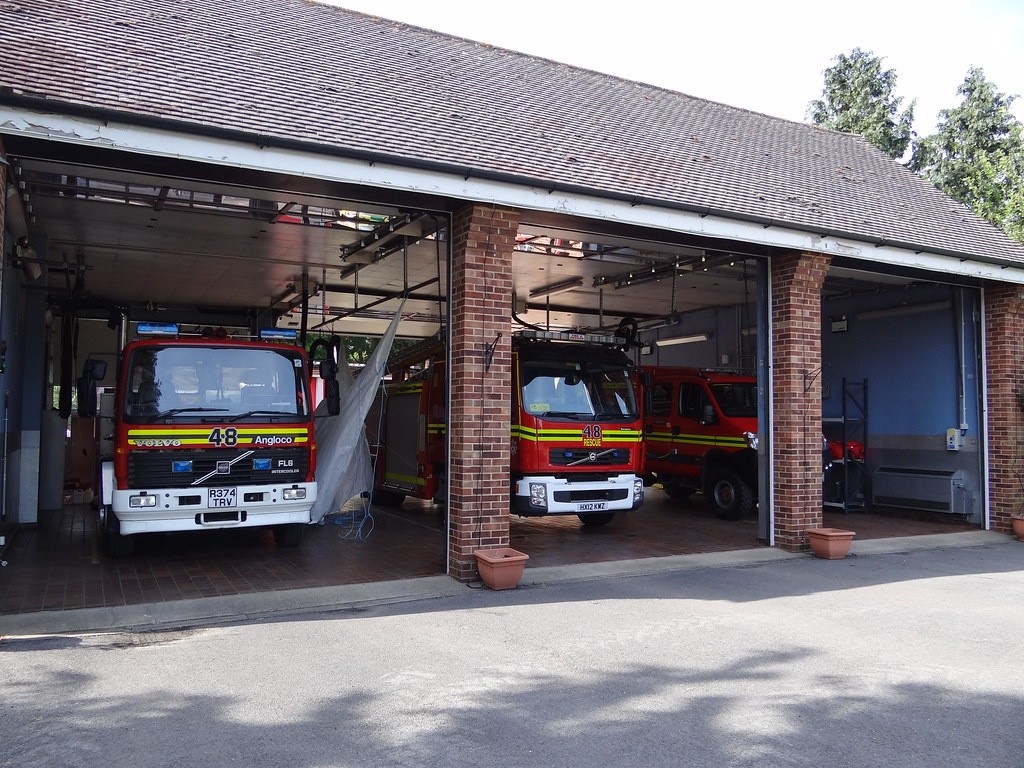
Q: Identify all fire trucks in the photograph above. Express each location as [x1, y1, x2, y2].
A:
[77, 323, 341, 559]
[352, 316, 643, 527]
[600, 364, 832, 521]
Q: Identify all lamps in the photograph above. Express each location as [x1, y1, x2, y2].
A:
[30, 216, 35, 224]
[529, 281, 582, 298]
[601, 255, 735, 285]
[15, 166, 21, 176]
[12, 234, 29, 268]
[19, 180, 27, 191]
[26, 204, 33, 213]
[344, 214, 412, 254]
[24, 193, 31, 203]
[341, 263, 368, 282]
[826, 313, 848, 334]
[655, 333, 709, 346]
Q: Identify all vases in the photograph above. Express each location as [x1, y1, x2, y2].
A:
[806, 528, 855, 558]
[473, 546, 529, 591]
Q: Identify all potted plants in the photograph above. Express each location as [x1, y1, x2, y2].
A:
[1011, 389, 1024, 542]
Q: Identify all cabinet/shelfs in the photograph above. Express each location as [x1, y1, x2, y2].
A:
[822, 379, 868, 512]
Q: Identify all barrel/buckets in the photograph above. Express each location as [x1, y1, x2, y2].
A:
[63, 488, 94, 505]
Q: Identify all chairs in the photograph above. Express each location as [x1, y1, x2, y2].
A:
[239, 368, 278, 403]
[139, 367, 181, 408]
[522, 364, 542, 406]
